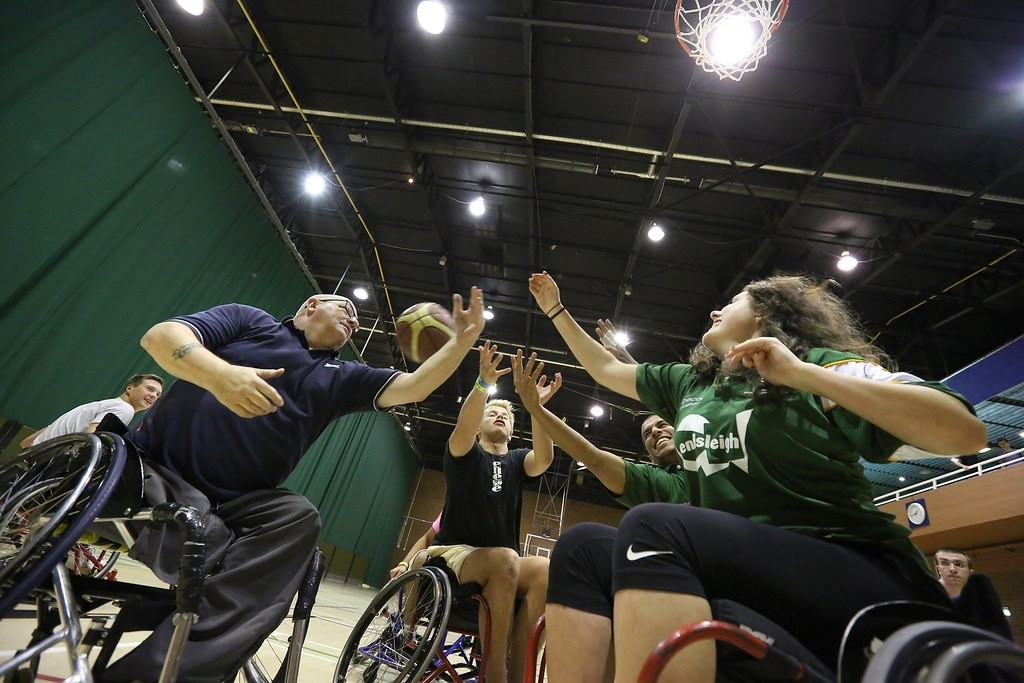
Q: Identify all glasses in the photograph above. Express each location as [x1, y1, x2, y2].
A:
[305, 298, 360, 333]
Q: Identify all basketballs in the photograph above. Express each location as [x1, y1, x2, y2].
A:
[395, 301, 457, 365]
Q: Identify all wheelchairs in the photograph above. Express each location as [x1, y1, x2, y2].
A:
[523, 593, 1024, 683]
[332, 555, 512, 683]
[0, 412, 325, 683]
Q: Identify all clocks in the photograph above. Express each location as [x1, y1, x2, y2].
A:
[907, 503, 926, 525]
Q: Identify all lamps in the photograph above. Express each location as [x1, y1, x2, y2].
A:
[469, 192, 485, 216]
[837, 248, 858, 271]
[647, 222, 665, 241]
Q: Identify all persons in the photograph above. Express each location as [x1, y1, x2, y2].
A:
[998, 438, 1024, 468]
[596, 317, 638, 365]
[93, 287, 484, 683]
[390, 511, 443, 642]
[951, 455, 979, 479]
[20, 374, 164, 449]
[528, 272, 988, 683]
[509, 348, 690, 510]
[427, 340, 563, 683]
[934, 548, 1014, 682]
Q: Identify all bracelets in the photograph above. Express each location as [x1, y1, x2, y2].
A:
[474, 377, 494, 393]
[543, 302, 564, 319]
[398, 562, 408, 568]
